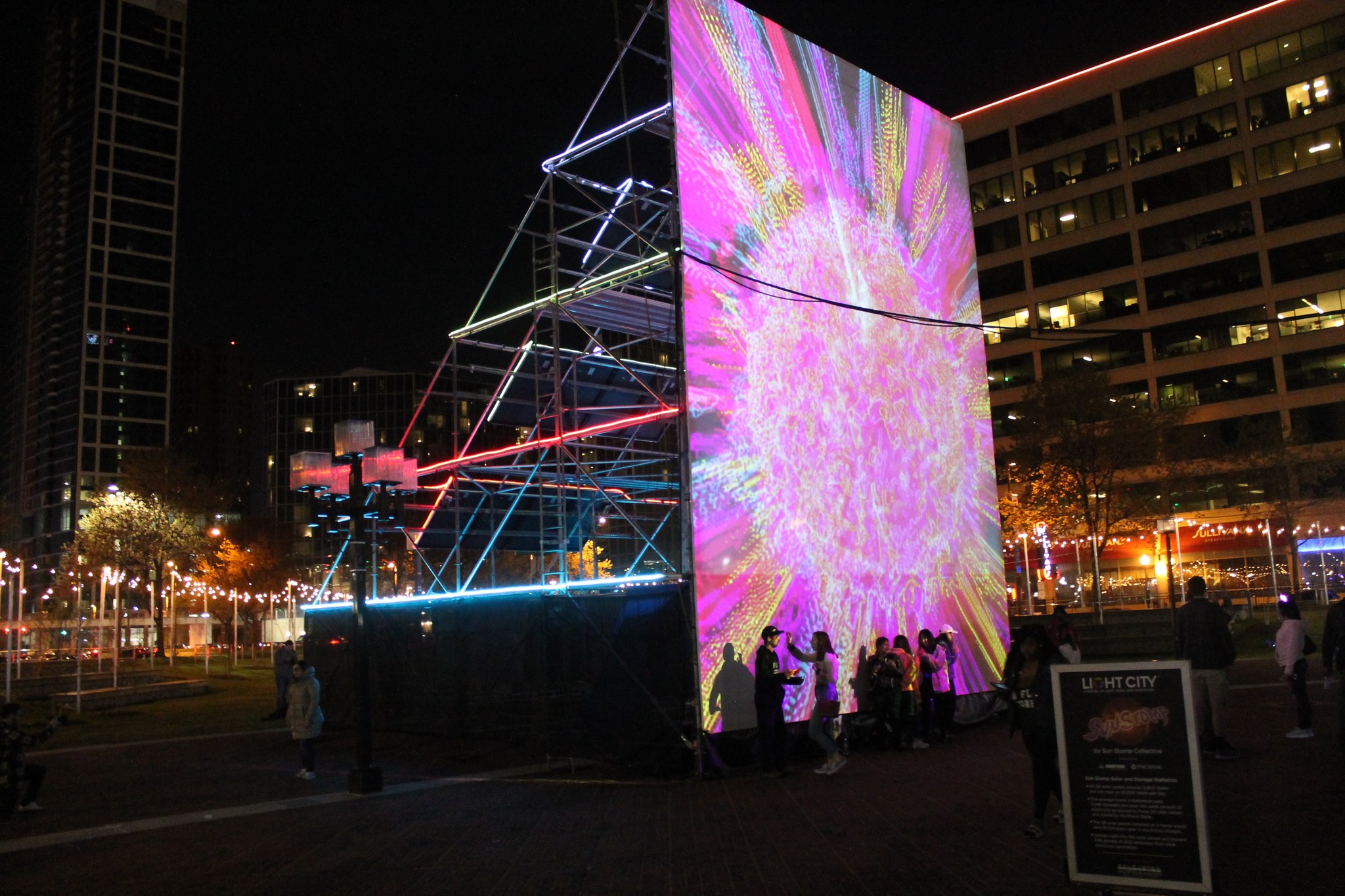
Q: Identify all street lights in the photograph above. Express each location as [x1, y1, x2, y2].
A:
[290, 420, 419, 794]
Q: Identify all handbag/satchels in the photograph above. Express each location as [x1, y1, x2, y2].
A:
[1301, 635, 1317, 655]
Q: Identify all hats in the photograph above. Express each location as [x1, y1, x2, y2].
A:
[939, 624, 958, 634]
[762, 626, 783, 639]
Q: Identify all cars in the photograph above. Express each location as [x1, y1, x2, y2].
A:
[1279, 590, 1338, 602]
[119, 649, 151, 659]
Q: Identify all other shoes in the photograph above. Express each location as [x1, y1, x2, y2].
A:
[815, 764, 827, 775]
[826, 754, 847, 775]
[1198, 740, 1212, 754]
[1053, 808, 1064, 825]
[912, 739, 929, 749]
[297, 769, 317, 781]
[1027, 823, 1044, 838]
[1212, 746, 1238, 758]
[1284, 726, 1314, 738]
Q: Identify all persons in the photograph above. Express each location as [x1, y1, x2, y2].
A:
[1273, 599, 1316, 738]
[284, 660, 323, 780]
[1322, 594, 1345, 696]
[1173, 576, 1242, 759]
[753, 605, 1082, 836]
[272, 640, 298, 723]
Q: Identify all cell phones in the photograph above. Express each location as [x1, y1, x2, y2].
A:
[990, 681, 1009, 689]
